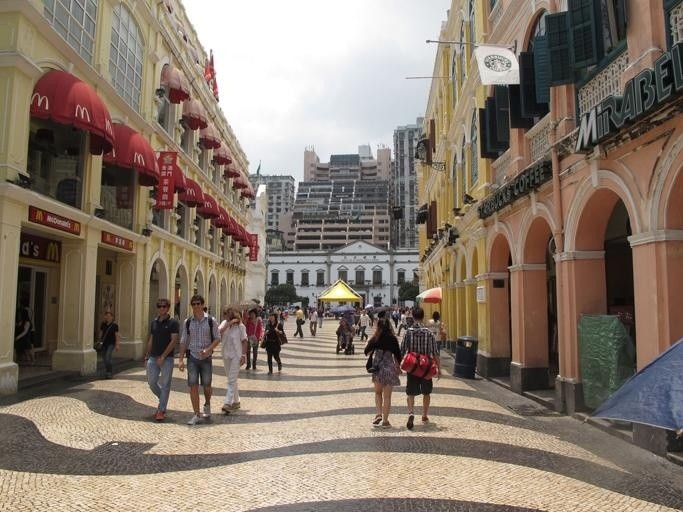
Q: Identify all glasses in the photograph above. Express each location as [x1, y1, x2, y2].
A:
[157, 305, 167, 308]
[191, 303, 200, 306]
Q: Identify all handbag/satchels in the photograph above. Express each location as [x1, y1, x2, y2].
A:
[400, 351, 437, 381]
[301, 321, 305, 325]
[365, 356, 380, 372]
[260, 334, 268, 349]
[440, 322, 448, 339]
[94, 338, 103, 352]
[248, 335, 258, 348]
[278, 331, 287, 345]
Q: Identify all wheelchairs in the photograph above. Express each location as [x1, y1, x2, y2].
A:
[335, 330, 355, 356]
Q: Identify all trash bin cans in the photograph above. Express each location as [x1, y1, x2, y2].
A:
[453, 336, 478, 379]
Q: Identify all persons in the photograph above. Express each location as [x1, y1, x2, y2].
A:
[396, 306, 413, 336]
[336, 306, 373, 355]
[425, 312, 447, 360]
[242, 303, 288, 376]
[98, 312, 120, 379]
[394, 307, 441, 429]
[145, 294, 249, 425]
[14, 303, 36, 367]
[363, 311, 401, 428]
[292, 306, 324, 338]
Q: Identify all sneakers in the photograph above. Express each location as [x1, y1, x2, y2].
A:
[106, 376, 112, 379]
[407, 412, 414, 429]
[233, 402, 241, 409]
[246, 364, 282, 376]
[382, 421, 391, 428]
[155, 410, 164, 420]
[422, 416, 429, 423]
[222, 404, 234, 412]
[187, 415, 202, 425]
[203, 403, 212, 418]
[373, 414, 382, 424]
